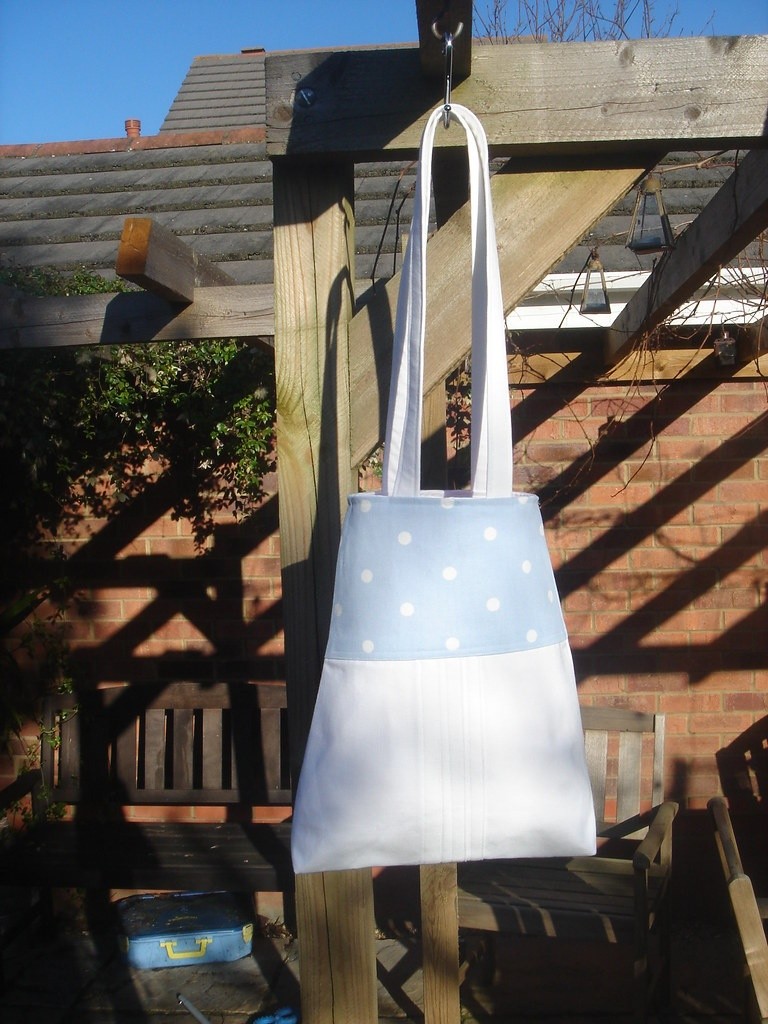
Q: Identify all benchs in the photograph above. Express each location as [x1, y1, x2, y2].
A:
[0, 665, 294, 953]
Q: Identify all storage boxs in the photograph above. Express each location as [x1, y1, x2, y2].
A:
[115, 890, 257, 969]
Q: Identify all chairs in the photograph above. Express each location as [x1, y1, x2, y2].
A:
[457, 704, 679, 1024]
[707, 795, 767, 1024]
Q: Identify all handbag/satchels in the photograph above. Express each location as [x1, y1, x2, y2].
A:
[290, 104, 598, 874]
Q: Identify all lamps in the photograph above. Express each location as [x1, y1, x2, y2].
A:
[578, 249, 611, 314]
[625, 167, 676, 255]
